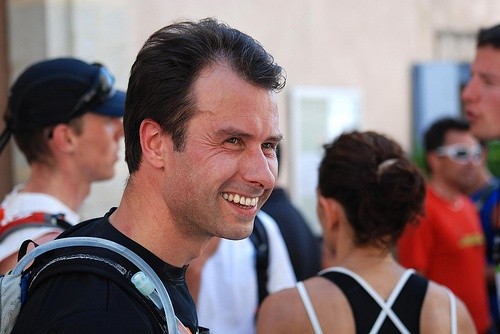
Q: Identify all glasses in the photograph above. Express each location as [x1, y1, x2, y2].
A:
[436, 142, 484, 167]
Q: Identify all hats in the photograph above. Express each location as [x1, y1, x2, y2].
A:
[4, 57, 126, 128]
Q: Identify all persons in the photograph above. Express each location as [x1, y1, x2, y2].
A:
[0, 17, 286, 334]
[0, 57, 126, 275]
[194, 26, 500, 334]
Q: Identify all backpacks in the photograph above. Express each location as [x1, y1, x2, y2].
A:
[0, 239, 190, 334]
